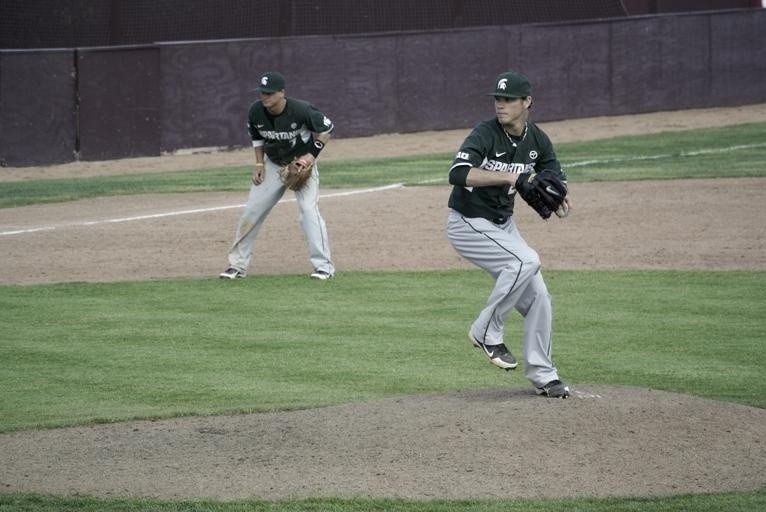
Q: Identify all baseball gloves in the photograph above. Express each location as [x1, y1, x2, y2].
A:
[278, 156, 314, 192]
[515, 169, 567, 219]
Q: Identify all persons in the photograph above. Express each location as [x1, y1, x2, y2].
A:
[218, 70, 335, 280]
[446, 70, 572, 399]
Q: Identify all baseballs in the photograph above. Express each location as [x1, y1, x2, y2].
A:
[555, 202, 569, 217]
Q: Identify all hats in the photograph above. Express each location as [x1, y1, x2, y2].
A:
[484, 70, 535, 100]
[251, 71, 287, 96]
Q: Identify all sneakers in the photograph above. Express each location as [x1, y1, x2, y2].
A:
[533, 376, 571, 400]
[219, 267, 248, 279]
[310, 267, 335, 280]
[467, 324, 519, 371]
